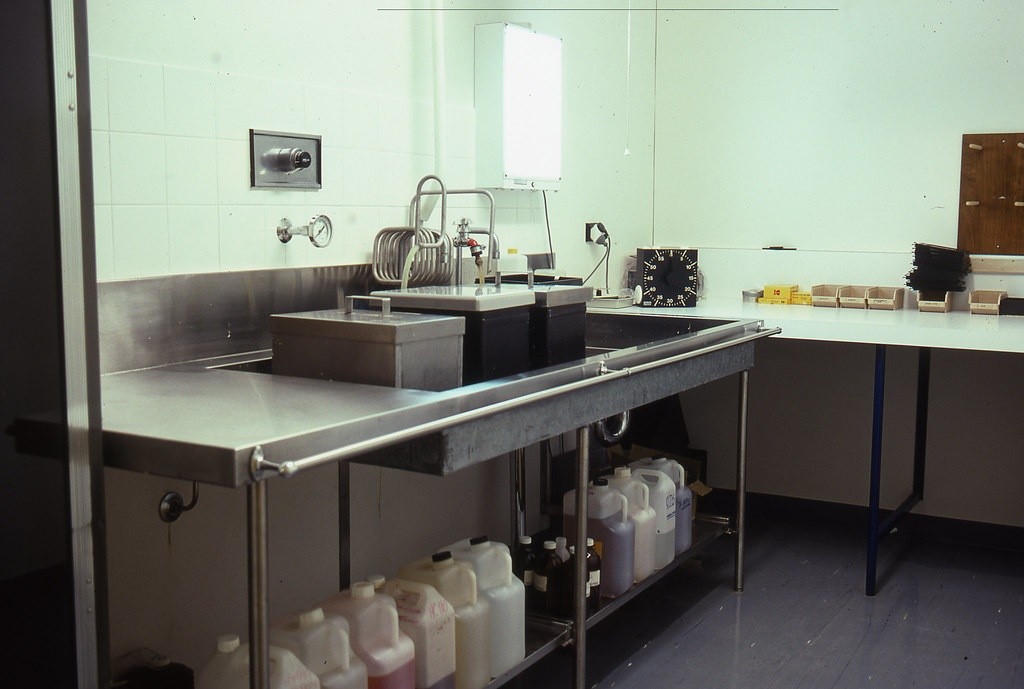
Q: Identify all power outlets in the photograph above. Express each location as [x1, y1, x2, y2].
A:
[586, 222, 600, 242]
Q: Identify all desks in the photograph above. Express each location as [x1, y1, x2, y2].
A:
[584, 297, 1023, 598]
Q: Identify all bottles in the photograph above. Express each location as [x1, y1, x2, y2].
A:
[556, 537, 570, 561]
[645, 458, 692, 556]
[512, 536, 535, 586]
[270, 608, 368, 689]
[533, 541, 562, 602]
[198, 634, 320, 689]
[632, 469, 676, 570]
[591, 467, 656, 582]
[437, 535, 525, 677]
[587, 538, 601, 609]
[398, 551, 491, 689]
[555, 545, 590, 621]
[313, 581, 416, 689]
[564, 478, 635, 599]
[367, 575, 456, 689]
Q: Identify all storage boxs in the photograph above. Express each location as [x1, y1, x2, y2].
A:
[368, 286, 536, 387]
[480, 283, 594, 368]
